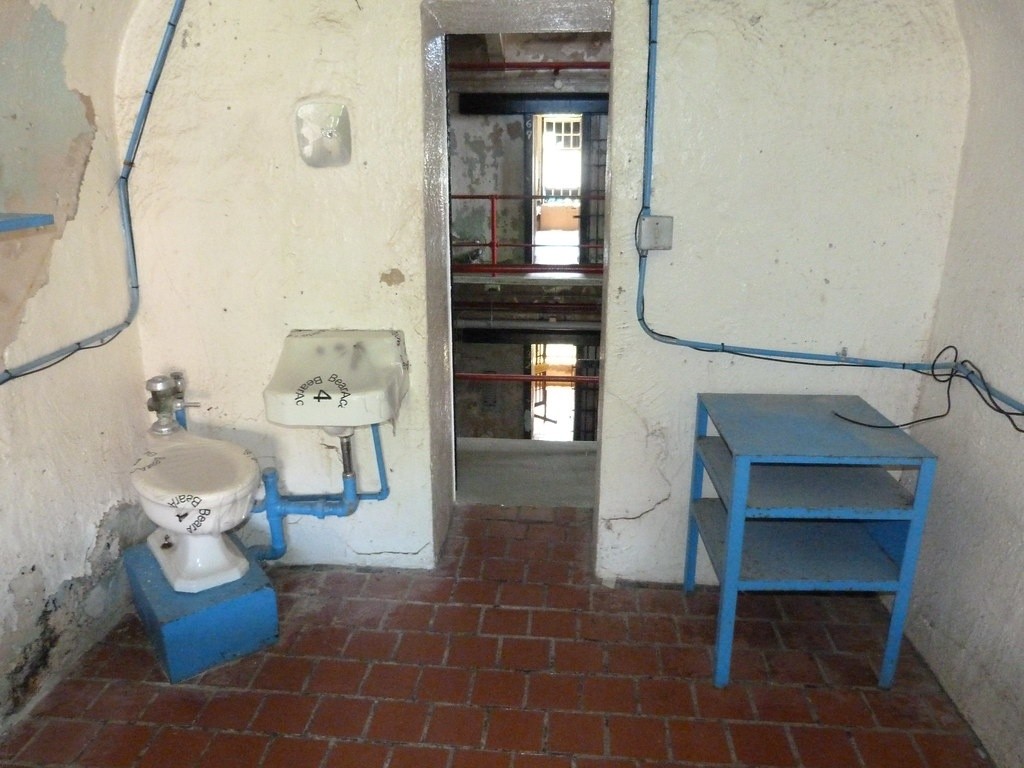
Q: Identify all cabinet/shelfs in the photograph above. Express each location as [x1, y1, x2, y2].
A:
[681, 393, 938, 688]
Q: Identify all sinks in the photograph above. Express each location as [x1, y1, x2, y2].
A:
[129, 424, 266, 593]
[263, 329, 410, 428]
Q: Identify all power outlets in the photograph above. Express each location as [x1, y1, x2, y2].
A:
[639, 214, 674, 252]
